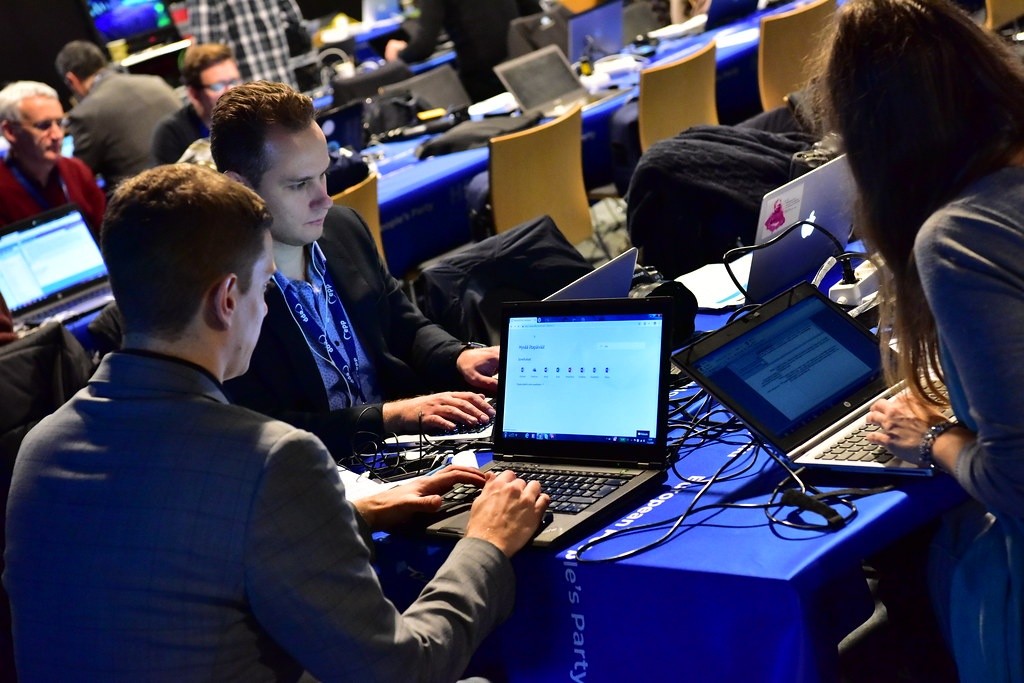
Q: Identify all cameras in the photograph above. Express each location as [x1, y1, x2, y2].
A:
[627, 265, 700, 350]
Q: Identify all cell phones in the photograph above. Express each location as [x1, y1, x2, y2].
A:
[369, 456, 444, 484]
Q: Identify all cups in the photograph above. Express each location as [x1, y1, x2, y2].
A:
[106, 39, 128, 62]
[335, 60, 355, 80]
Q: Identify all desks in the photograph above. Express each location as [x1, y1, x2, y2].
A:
[0, 0, 968, 683]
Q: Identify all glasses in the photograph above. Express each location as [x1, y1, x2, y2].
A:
[190, 77, 245, 95]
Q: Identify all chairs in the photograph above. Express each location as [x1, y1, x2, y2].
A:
[0, 0, 1023, 510]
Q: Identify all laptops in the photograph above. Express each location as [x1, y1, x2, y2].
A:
[314, 0, 760, 153]
[385, 153, 962, 556]
[60, 133, 109, 187]
[0, 205, 119, 326]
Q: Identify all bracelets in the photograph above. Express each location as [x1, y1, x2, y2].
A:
[918, 420, 959, 470]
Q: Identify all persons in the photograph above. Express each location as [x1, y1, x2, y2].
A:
[386, 0, 544, 113]
[152, 44, 244, 168]
[188, 0, 308, 99]
[196, 81, 505, 452]
[825, 0, 1024, 683]
[0, 80, 107, 338]
[0, 165, 552, 683]
[59, 41, 186, 196]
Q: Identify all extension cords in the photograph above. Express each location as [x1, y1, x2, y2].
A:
[829, 251, 890, 305]
